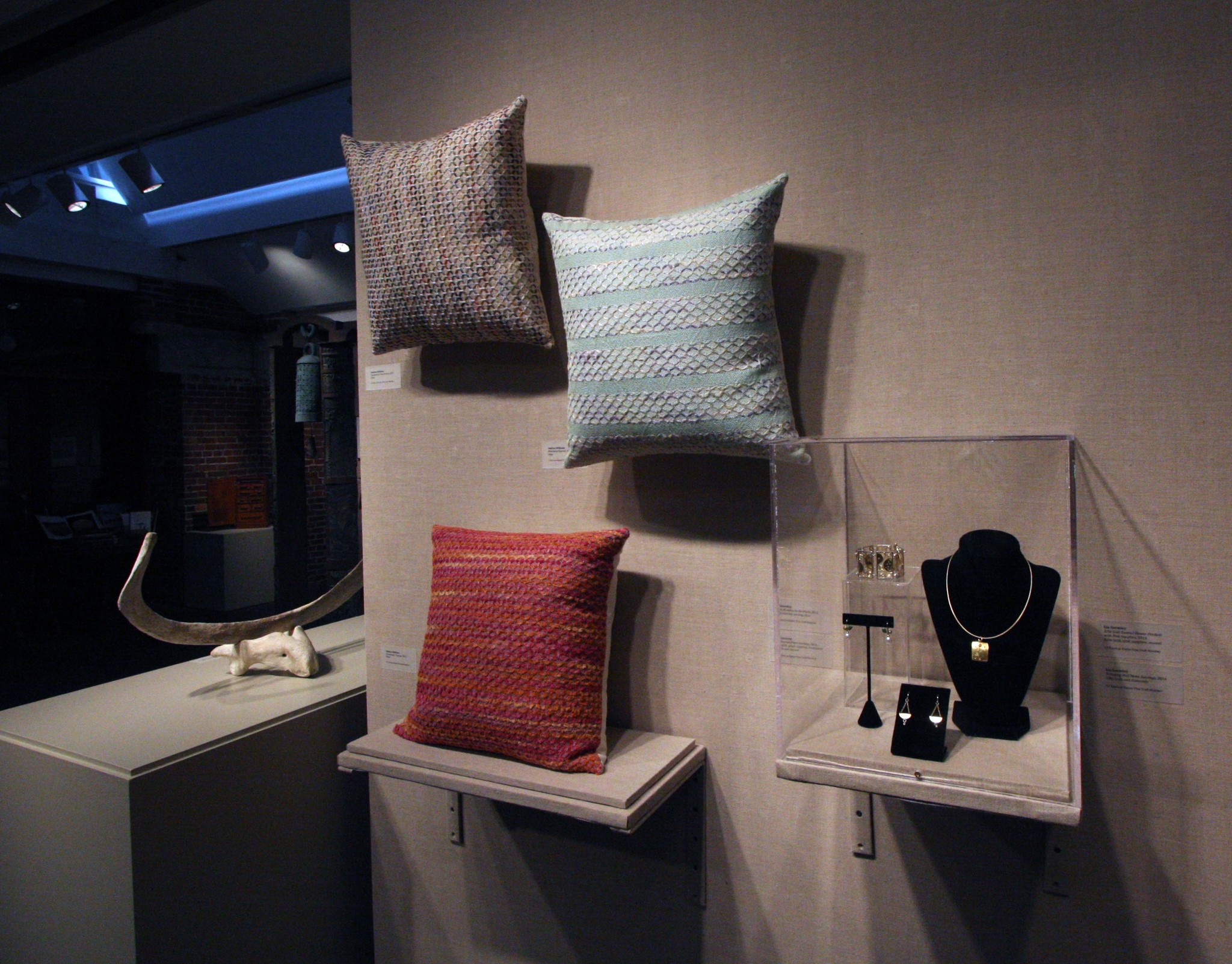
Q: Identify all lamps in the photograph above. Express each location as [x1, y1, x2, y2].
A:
[292, 221, 313, 259]
[118, 148, 164, 194]
[243, 234, 269, 274]
[0, 184, 25, 227]
[333, 216, 351, 254]
[45, 168, 90, 213]
[5, 177, 44, 219]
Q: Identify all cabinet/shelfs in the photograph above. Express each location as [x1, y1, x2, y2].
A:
[0, 609, 371, 964]
[760, 432, 1084, 830]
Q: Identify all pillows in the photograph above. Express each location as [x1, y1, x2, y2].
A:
[339, 99, 556, 352]
[392, 526, 633, 773]
[541, 171, 810, 472]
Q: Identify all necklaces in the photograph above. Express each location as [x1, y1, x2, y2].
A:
[946, 552, 1034, 662]
